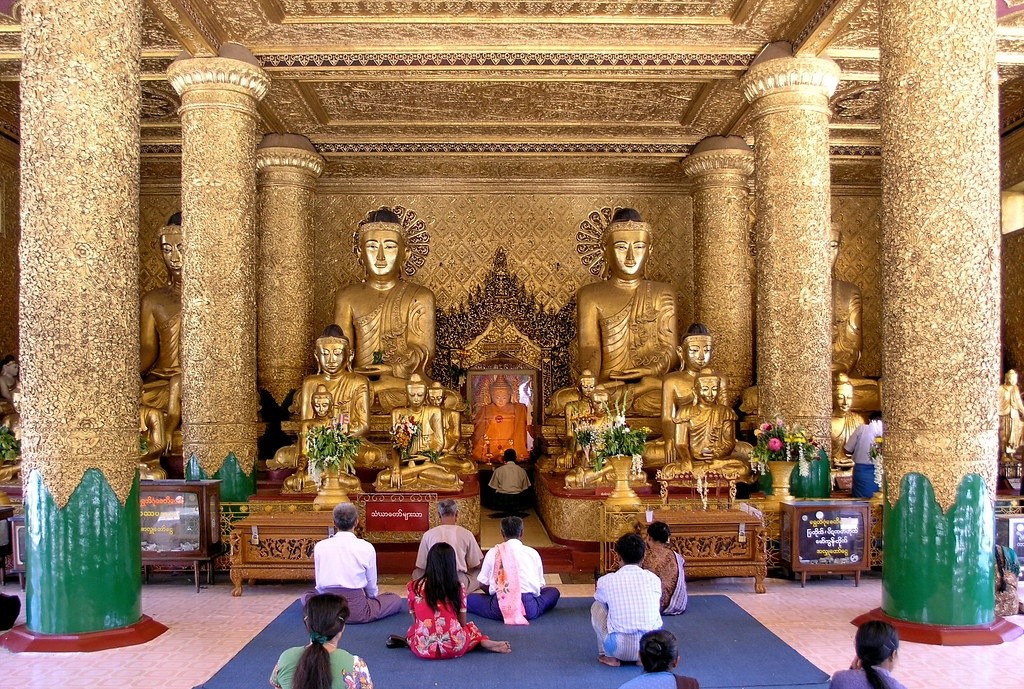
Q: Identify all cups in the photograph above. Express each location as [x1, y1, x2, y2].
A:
[645, 511, 653, 523]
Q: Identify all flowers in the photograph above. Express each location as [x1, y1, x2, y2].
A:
[306, 413, 366, 490]
[867, 435, 884, 490]
[570, 391, 653, 479]
[388, 413, 422, 460]
[746, 417, 822, 478]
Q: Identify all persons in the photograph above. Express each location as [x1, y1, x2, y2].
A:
[473, 373, 529, 462]
[829, 372, 865, 490]
[740, 219, 879, 414]
[0, 353, 19, 423]
[466, 516, 559, 626]
[266, 205, 474, 496]
[0, 385, 22, 485]
[393, 542, 512, 658]
[545, 207, 756, 489]
[412, 498, 483, 595]
[589, 533, 663, 668]
[998, 369, 1024, 455]
[829, 619, 906, 689]
[844, 409, 883, 498]
[617, 629, 701, 689]
[138, 208, 182, 453]
[138, 373, 168, 481]
[299, 502, 403, 623]
[268, 593, 375, 689]
[641, 521, 688, 615]
[486, 448, 533, 512]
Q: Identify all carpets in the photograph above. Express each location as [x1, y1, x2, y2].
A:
[193, 594, 836, 689]
